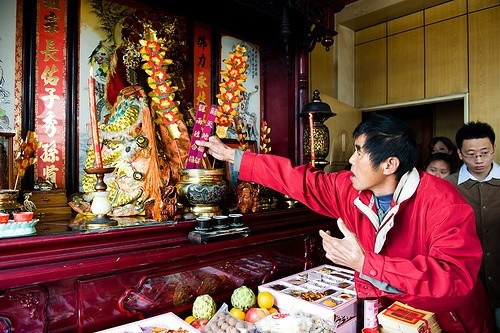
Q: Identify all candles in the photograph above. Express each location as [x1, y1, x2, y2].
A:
[308, 113, 315, 168]
[88, 57, 104, 168]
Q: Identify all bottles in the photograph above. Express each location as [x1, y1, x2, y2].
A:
[357, 298, 378, 333]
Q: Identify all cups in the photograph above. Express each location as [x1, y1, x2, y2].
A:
[195, 214, 244, 231]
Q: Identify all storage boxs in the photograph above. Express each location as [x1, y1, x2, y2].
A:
[258, 264, 357, 328]
[332, 317, 357, 333]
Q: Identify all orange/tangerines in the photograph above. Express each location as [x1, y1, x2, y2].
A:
[230, 307, 246, 321]
[257, 292, 278, 314]
[185, 316, 196, 325]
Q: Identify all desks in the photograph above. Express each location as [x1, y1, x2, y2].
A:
[0, 204, 353, 333]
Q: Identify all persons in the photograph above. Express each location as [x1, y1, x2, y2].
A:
[425, 120, 500, 333]
[195, 114, 494, 333]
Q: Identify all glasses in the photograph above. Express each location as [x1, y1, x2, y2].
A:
[460, 146, 495, 159]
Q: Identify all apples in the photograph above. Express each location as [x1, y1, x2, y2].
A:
[244, 307, 270, 323]
